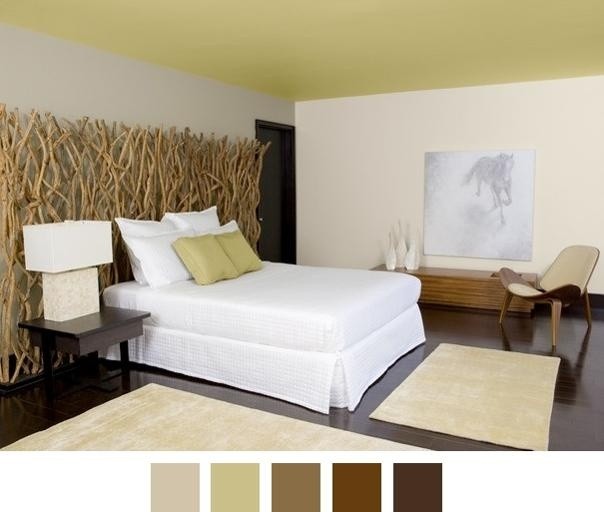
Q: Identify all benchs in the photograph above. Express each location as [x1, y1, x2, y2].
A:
[369, 263, 537, 314]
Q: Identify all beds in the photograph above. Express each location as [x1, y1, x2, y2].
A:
[105, 260, 426, 415]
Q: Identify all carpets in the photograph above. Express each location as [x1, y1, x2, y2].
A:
[0, 382, 437, 451]
[367, 344, 562, 450]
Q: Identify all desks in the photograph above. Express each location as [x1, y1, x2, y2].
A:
[18, 304, 150, 411]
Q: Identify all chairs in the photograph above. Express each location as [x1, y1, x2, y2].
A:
[495, 243, 600, 346]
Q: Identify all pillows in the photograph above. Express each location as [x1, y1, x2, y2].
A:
[112, 205, 264, 288]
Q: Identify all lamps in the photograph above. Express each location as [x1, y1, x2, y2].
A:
[22, 220, 114, 324]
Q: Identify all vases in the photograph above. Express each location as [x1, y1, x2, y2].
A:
[385, 218, 422, 270]
